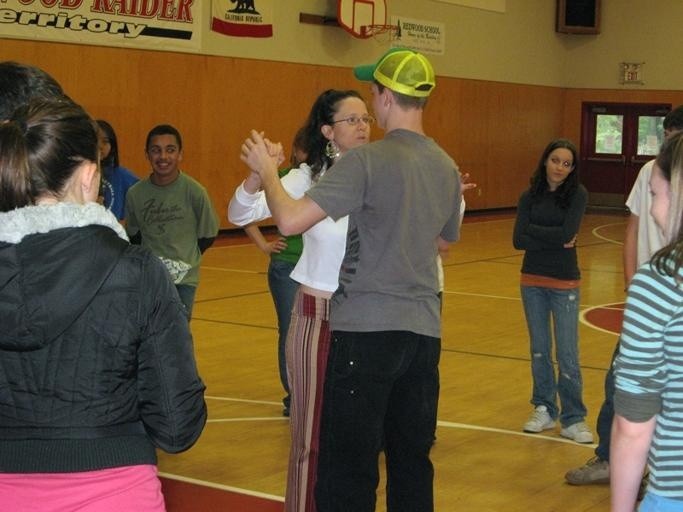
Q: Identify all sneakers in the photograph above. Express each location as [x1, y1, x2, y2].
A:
[524, 405, 594, 443]
[565, 456, 609, 485]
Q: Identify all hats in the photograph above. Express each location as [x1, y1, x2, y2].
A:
[354, 48, 435, 98]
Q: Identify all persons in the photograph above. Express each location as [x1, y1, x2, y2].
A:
[0, 62, 62, 117]
[125, 125, 218, 321]
[513, 139, 594, 444]
[564, 106, 683, 486]
[608, 130, 683, 512]
[93, 120, 139, 225]
[227, 89, 478, 512]
[1, 96, 207, 512]
[239, 46, 462, 512]
[242, 126, 307, 417]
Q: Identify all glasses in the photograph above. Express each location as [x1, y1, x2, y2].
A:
[333, 115, 376, 126]
